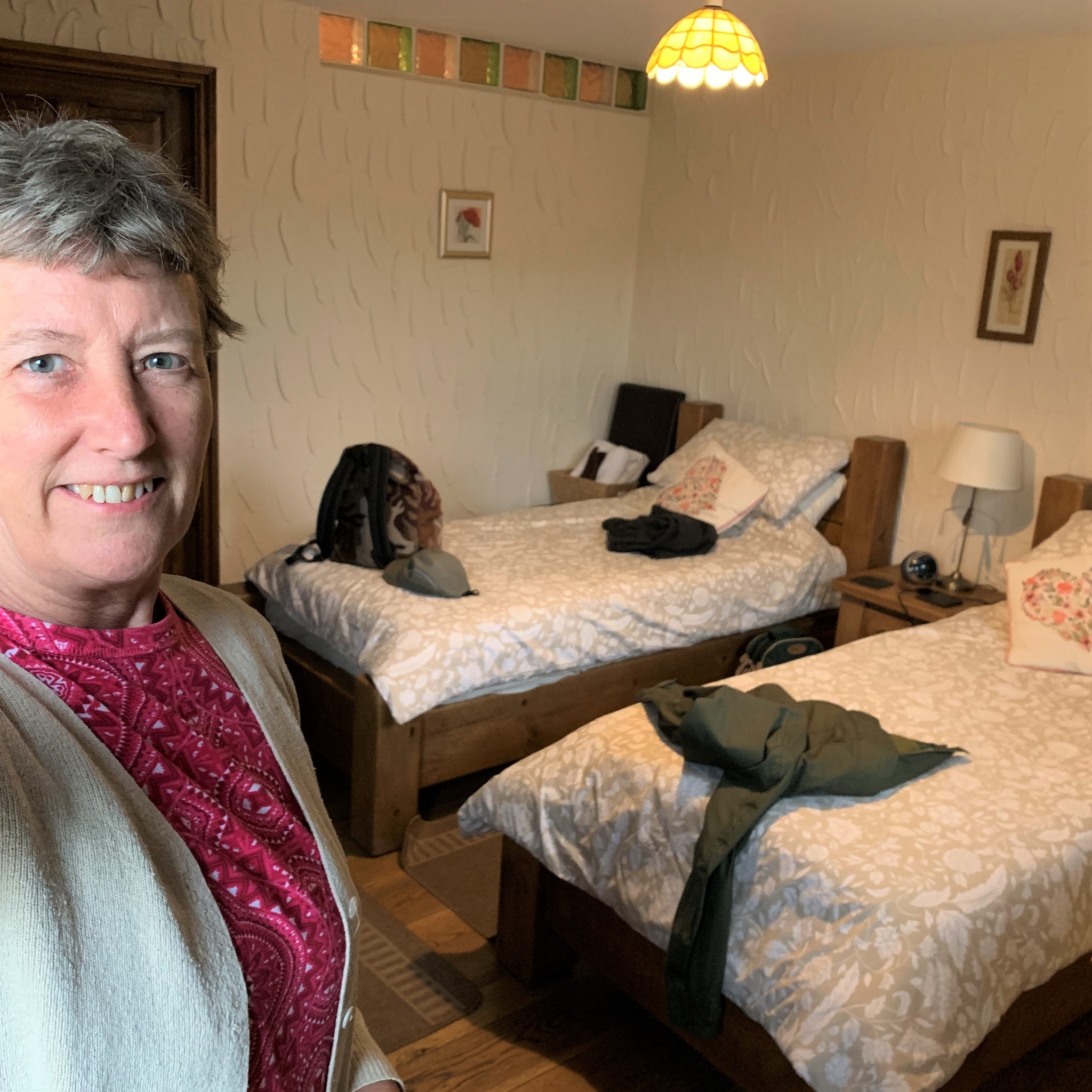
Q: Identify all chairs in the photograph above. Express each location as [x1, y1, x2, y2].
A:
[240, 399, 906, 858]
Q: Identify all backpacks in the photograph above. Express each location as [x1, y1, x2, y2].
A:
[284, 443, 445, 567]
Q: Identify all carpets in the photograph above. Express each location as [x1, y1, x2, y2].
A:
[399, 811, 503, 948]
[350, 879, 485, 1055]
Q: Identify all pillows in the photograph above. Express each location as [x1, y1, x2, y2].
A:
[1023, 507, 1092, 560]
[646, 416, 856, 523]
[1003, 554, 1092, 677]
[653, 437, 768, 540]
[795, 472, 849, 526]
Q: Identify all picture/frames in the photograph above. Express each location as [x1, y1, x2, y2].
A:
[439, 189, 495, 259]
[976, 229, 1052, 343]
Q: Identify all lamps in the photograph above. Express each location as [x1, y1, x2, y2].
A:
[933, 422, 1023, 593]
[643, 0, 769, 93]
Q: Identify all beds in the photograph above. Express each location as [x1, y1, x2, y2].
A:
[455, 471, 1092, 1092]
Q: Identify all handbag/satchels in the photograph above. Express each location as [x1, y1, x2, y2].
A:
[734, 632, 824, 678]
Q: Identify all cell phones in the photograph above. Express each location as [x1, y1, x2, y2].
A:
[917, 591, 963, 609]
[848, 575, 893, 589]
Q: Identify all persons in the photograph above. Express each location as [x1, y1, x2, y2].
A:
[1, 101, 361, 1092]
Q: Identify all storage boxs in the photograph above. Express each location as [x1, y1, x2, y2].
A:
[548, 467, 640, 505]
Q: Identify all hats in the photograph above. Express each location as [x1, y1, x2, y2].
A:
[382, 547, 480, 599]
